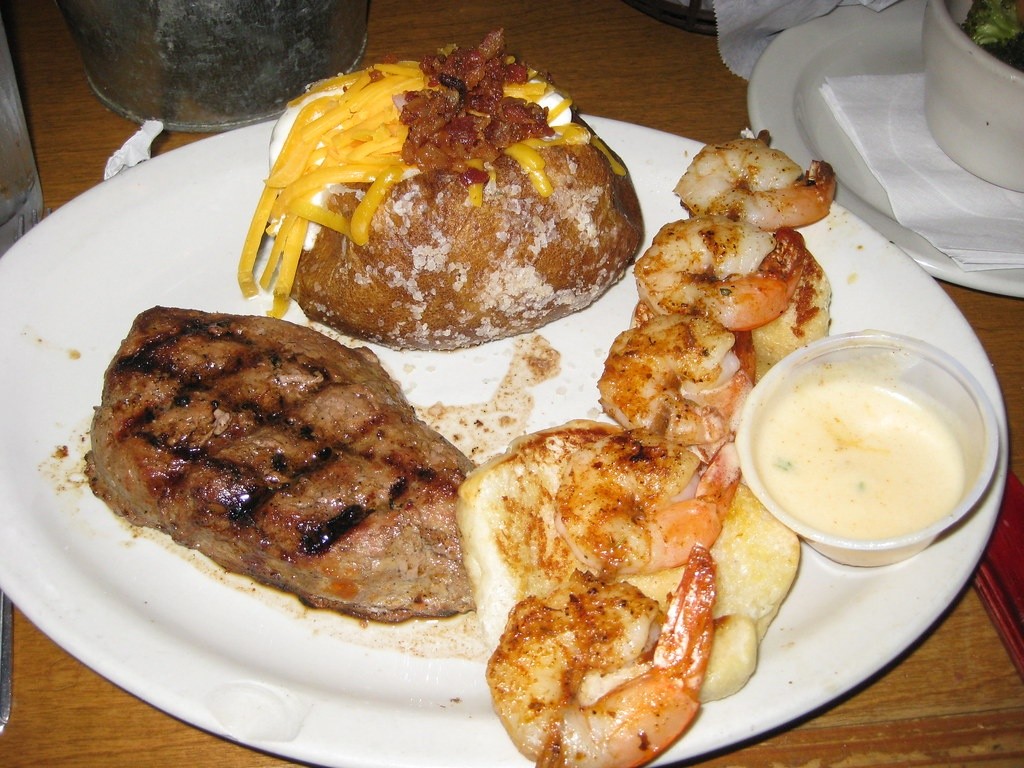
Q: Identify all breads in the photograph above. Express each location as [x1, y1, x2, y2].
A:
[290, 108, 644, 353]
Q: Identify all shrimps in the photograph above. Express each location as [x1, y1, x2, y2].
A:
[485, 137, 837, 768]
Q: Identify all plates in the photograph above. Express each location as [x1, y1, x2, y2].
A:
[745, 0, 1024, 300]
[1, 115, 1009, 768]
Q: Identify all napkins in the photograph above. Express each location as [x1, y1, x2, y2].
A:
[817, 71, 1024, 272]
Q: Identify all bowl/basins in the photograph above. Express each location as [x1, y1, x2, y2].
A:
[732, 330, 1000, 568]
[922, 0, 1024, 193]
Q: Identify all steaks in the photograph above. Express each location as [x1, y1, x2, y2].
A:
[87, 304, 483, 623]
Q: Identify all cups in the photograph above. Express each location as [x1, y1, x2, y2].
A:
[0, 18, 45, 256]
